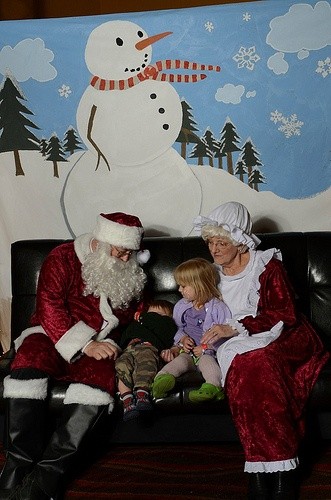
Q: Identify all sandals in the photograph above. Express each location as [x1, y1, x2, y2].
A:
[189, 383, 225, 402]
[153, 374, 175, 399]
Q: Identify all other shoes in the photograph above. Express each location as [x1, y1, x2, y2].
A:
[119, 385, 152, 423]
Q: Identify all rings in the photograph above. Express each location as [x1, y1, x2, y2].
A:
[208, 329, 211, 332]
[213, 332, 217, 336]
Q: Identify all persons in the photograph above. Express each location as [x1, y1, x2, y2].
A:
[152, 258, 232, 404]
[115, 299, 178, 423]
[161, 202, 331, 500]
[0, 212, 150, 500]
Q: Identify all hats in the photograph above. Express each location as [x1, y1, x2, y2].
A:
[194, 201, 262, 249]
[92, 212, 150, 264]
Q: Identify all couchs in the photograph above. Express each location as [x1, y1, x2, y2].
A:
[0, 232, 331, 445]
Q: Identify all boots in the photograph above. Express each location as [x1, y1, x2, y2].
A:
[0, 375, 48, 500]
[9, 383, 114, 500]
[244, 457, 300, 500]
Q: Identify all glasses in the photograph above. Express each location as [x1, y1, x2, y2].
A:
[207, 241, 229, 248]
[113, 245, 133, 256]
